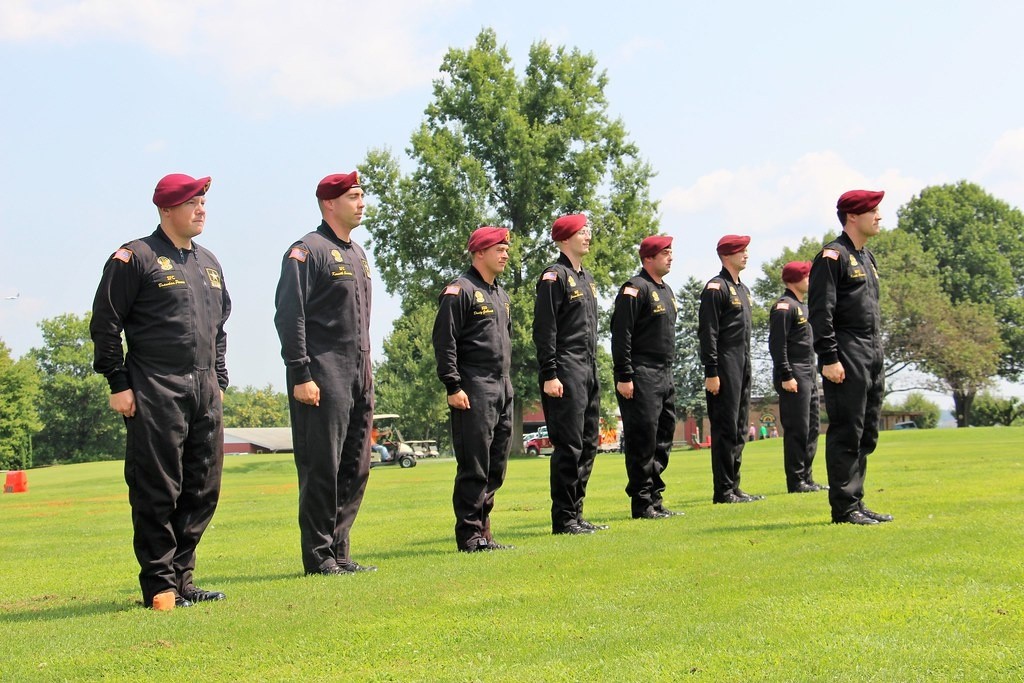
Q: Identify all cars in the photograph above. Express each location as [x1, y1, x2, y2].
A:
[893, 421, 917, 431]
[522, 425, 550, 444]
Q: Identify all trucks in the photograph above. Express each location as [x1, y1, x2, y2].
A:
[526, 415, 622, 457]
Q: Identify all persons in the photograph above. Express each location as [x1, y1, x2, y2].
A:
[371, 422, 393, 460]
[532, 214, 610, 536]
[619, 431, 625, 454]
[768, 261, 830, 494]
[806, 190, 896, 526]
[748, 423, 778, 442]
[274, 171, 375, 578]
[89, 174, 231, 607]
[611, 235, 686, 519]
[432, 227, 516, 553]
[697, 234, 765, 504]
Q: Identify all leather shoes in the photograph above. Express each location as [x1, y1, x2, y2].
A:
[186, 587, 225, 602]
[643, 510, 669, 518]
[724, 493, 754, 504]
[578, 517, 610, 530]
[657, 508, 685, 516]
[322, 564, 353, 574]
[835, 511, 879, 525]
[735, 490, 764, 500]
[339, 560, 377, 572]
[562, 525, 594, 533]
[860, 505, 894, 522]
[794, 483, 820, 493]
[175, 594, 193, 607]
[809, 483, 830, 490]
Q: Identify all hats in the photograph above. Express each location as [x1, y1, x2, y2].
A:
[639, 236, 673, 258]
[153, 173, 211, 208]
[316, 171, 360, 200]
[552, 214, 587, 241]
[836, 190, 885, 216]
[716, 235, 750, 256]
[468, 227, 510, 252]
[781, 261, 814, 285]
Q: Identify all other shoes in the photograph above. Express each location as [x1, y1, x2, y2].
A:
[471, 542, 515, 552]
